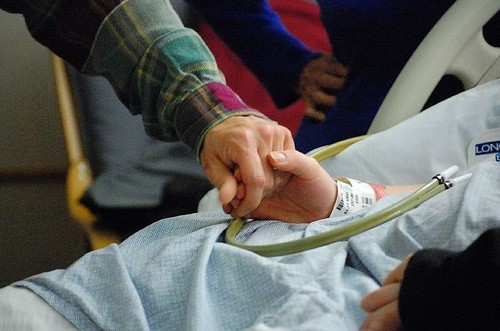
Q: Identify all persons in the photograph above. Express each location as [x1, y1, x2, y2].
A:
[0, 150, 500, 331]
[67, 0, 331, 245]
[0, 0, 295, 240]
[184, 0, 500, 157]
